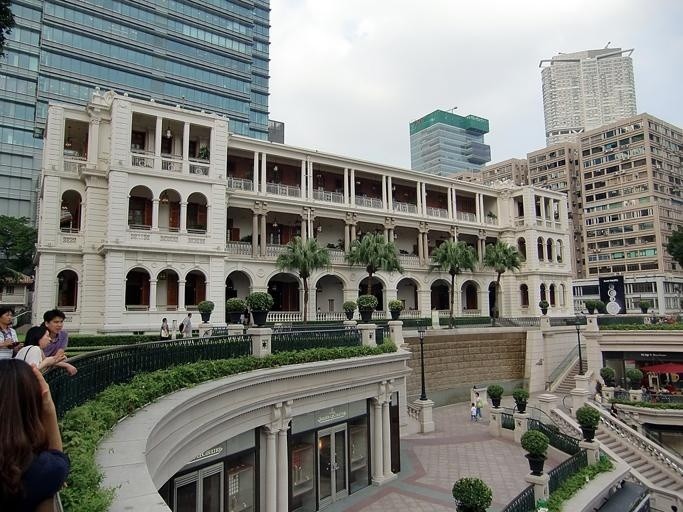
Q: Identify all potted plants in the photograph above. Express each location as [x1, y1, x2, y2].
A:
[600, 367, 644, 390]
[539, 300, 549, 315]
[451, 385, 600, 512]
[197, 291, 403, 328]
[585, 300, 650, 314]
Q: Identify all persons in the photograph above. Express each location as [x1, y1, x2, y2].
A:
[473, 392, 482, 420]
[39, 308, 79, 377]
[639, 381, 682, 403]
[0, 357, 71, 511]
[649, 310, 678, 324]
[469, 402, 477, 423]
[159, 317, 170, 348]
[14, 326, 67, 374]
[0, 305, 24, 360]
[317, 306, 321, 313]
[178, 312, 195, 346]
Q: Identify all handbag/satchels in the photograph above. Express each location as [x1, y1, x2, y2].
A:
[475, 401, 483, 408]
[160, 329, 169, 338]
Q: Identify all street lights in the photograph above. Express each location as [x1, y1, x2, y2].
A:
[417, 326, 428, 401]
[574, 319, 585, 375]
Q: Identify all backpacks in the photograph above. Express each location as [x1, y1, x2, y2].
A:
[178, 322, 184, 332]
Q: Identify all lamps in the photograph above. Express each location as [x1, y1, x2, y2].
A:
[162, 120, 173, 139]
[65, 125, 73, 146]
[161, 192, 169, 204]
[274, 160, 278, 171]
[272, 216, 278, 227]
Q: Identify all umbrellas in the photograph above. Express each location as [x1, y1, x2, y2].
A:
[639, 362, 683, 387]
